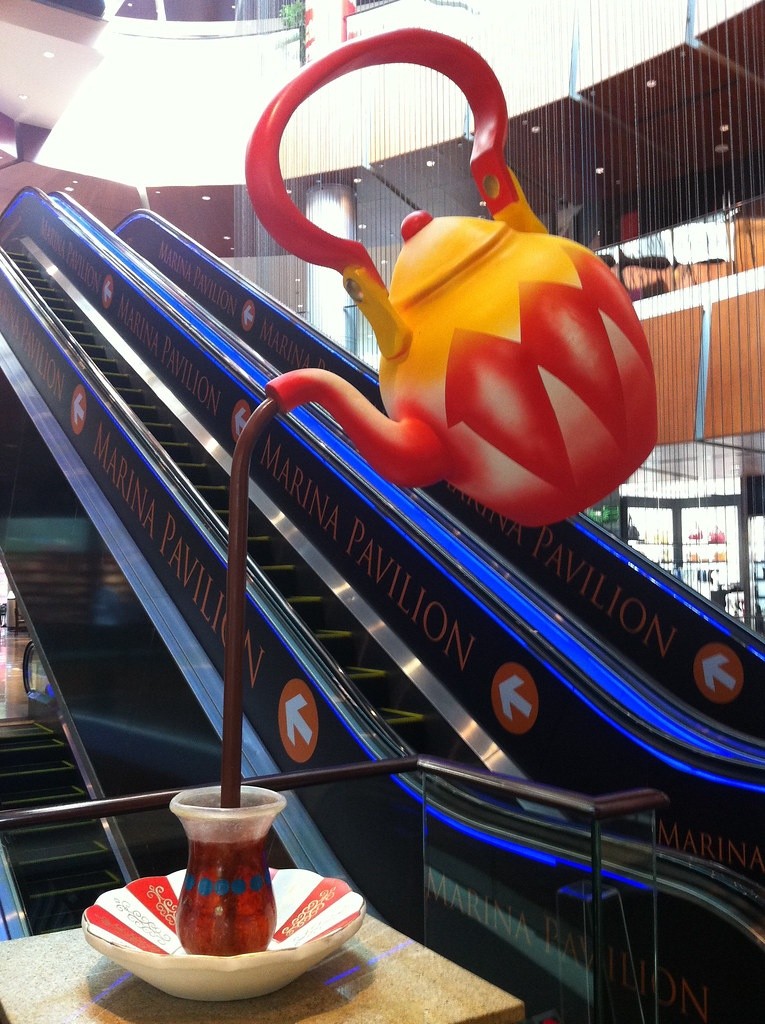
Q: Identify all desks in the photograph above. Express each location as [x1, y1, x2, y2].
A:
[0, 913, 525, 1024]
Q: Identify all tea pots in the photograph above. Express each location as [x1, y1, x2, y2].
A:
[245, 27, 657, 528]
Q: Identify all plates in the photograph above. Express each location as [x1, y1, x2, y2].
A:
[81, 863, 366, 1002]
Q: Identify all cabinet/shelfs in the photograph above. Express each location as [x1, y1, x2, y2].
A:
[636, 506, 729, 614]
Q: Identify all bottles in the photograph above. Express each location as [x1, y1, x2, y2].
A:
[170, 786, 287, 955]
